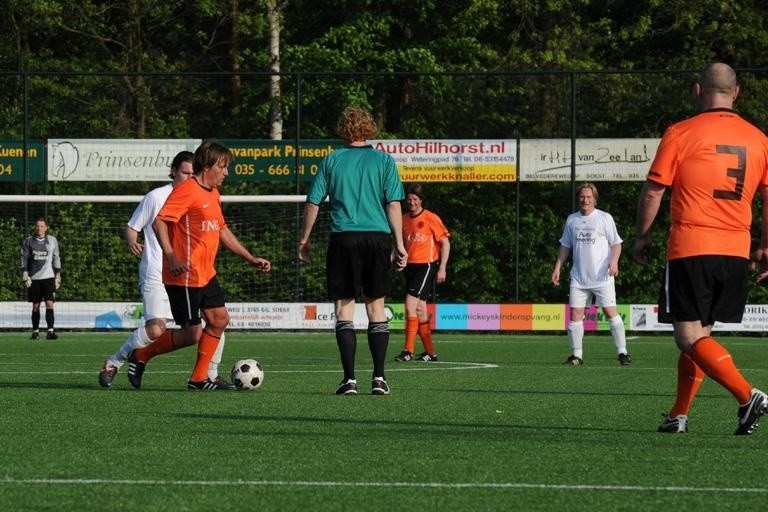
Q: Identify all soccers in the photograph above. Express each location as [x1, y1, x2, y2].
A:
[231, 358, 264, 390]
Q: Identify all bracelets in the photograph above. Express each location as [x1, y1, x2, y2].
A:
[300, 239, 309, 246]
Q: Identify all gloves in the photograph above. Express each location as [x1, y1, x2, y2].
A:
[54, 274, 61, 289]
[22, 271, 31, 288]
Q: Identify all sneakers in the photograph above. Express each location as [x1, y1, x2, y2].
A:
[617, 353, 632, 365]
[46, 331, 58, 340]
[415, 352, 438, 362]
[99, 359, 118, 387]
[187, 378, 229, 390]
[394, 350, 416, 362]
[370, 376, 390, 395]
[335, 379, 357, 395]
[213, 375, 236, 388]
[656, 413, 689, 434]
[563, 355, 583, 365]
[31, 332, 40, 339]
[127, 348, 145, 388]
[733, 388, 767, 434]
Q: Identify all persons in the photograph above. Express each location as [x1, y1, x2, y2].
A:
[551, 183, 632, 366]
[20, 219, 62, 340]
[127, 142, 271, 390]
[633, 62, 768, 436]
[389, 182, 451, 362]
[98, 154, 236, 391]
[298, 105, 408, 394]
